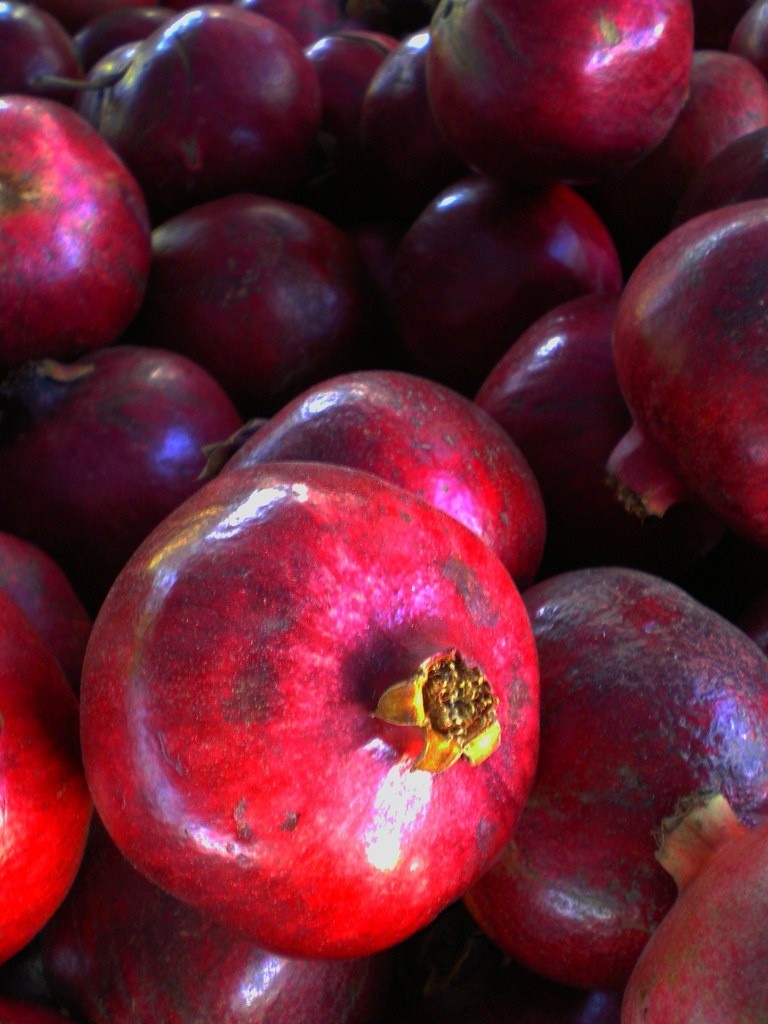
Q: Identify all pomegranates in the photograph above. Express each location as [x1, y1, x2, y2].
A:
[0, 0, 768, 1024]
